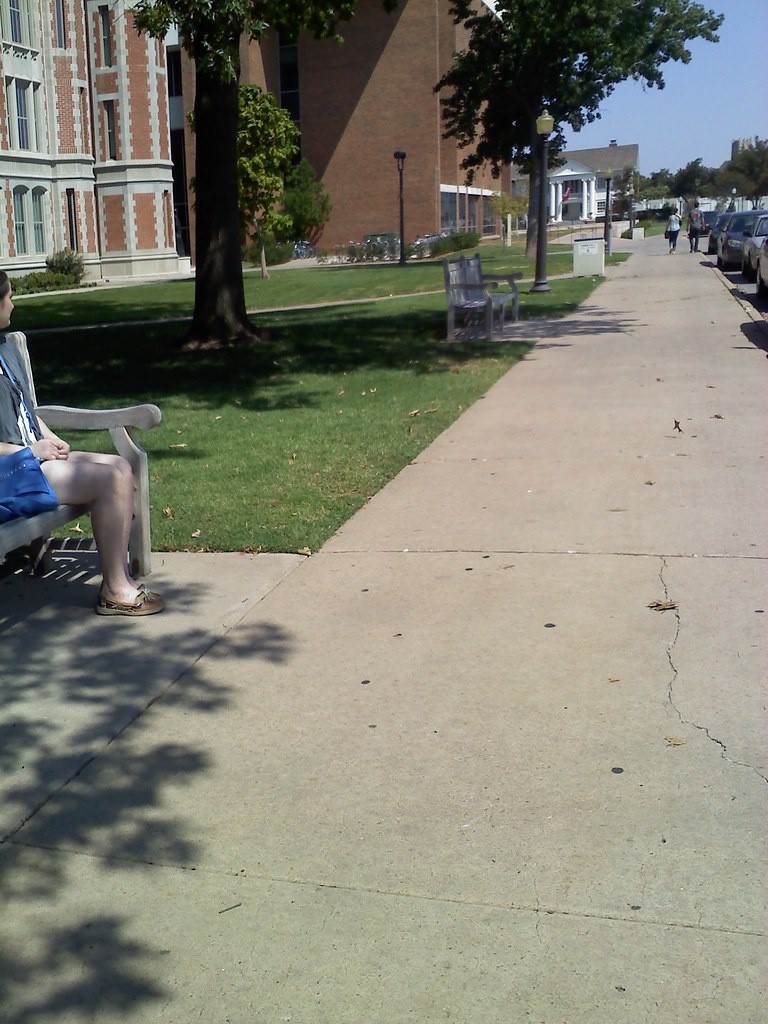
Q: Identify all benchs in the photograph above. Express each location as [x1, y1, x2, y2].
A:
[443, 253, 523, 340]
[0, 331, 162, 580]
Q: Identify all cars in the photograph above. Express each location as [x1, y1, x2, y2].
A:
[716, 210, 768, 270]
[741, 213, 768, 280]
[700, 210, 724, 236]
[755, 234, 768, 298]
[706, 211, 735, 254]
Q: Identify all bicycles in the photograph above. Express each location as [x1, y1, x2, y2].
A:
[272, 231, 436, 265]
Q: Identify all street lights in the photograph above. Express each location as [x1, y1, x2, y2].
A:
[604, 167, 615, 256]
[732, 187, 737, 210]
[393, 149, 407, 263]
[528, 109, 554, 293]
[629, 187, 636, 228]
[677, 195, 683, 215]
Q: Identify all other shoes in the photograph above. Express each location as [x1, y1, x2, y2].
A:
[95, 580, 165, 615]
[694, 249, 701, 252]
[669, 249, 676, 254]
[690, 250, 694, 253]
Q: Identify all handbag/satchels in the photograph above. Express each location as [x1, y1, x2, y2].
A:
[664, 231, 670, 239]
[0, 447, 59, 524]
[679, 220, 682, 226]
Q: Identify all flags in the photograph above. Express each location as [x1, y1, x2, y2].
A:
[563, 187, 571, 202]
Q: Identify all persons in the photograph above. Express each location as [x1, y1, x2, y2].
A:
[0, 269, 165, 616]
[666, 209, 682, 254]
[686, 202, 705, 253]
